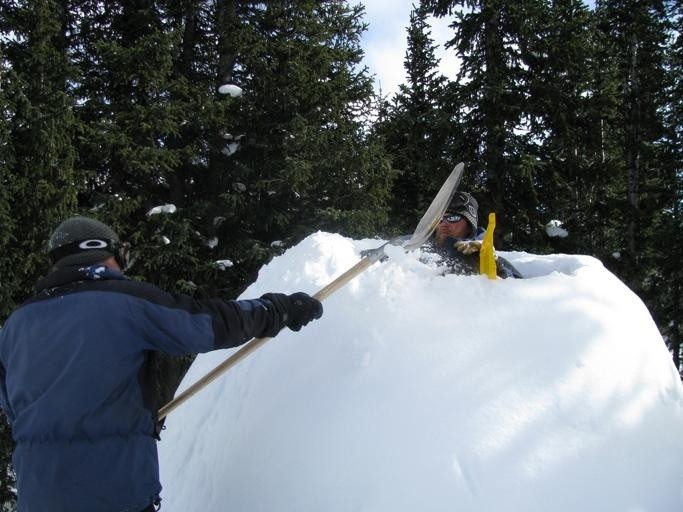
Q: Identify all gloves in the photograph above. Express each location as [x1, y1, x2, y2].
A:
[283, 292, 324, 332]
[453, 239, 497, 260]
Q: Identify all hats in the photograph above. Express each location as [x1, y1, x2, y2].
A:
[446, 190, 480, 237]
[46, 217, 133, 270]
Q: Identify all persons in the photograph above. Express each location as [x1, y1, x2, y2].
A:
[0, 216, 321, 512]
[361, 189, 521, 278]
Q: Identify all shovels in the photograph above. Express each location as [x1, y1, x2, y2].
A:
[156, 161, 465, 422]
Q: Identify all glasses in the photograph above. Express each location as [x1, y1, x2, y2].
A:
[441, 214, 461, 224]
[108, 240, 135, 269]
